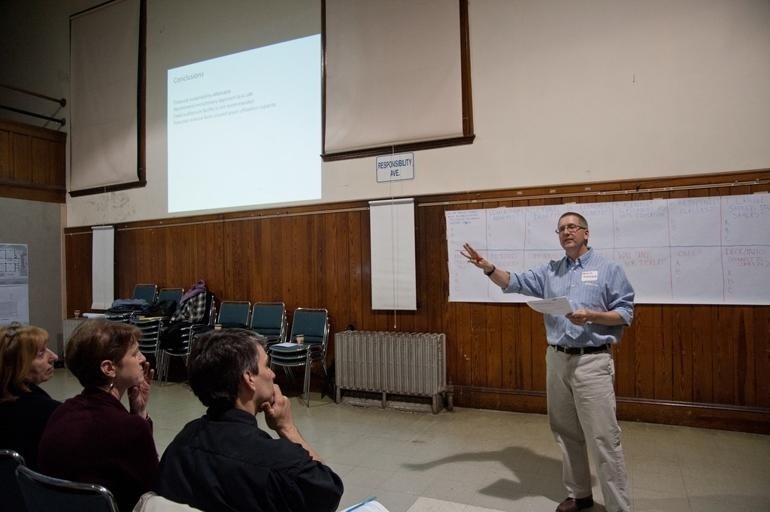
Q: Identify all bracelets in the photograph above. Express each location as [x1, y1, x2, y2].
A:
[483, 264, 496, 276]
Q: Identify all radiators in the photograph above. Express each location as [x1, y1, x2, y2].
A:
[331, 328, 451, 412]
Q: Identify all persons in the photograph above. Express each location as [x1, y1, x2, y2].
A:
[34, 318, 161, 511]
[0, 320, 65, 470]
[156, 328, 344, 512]
[458, 210, 636, 511]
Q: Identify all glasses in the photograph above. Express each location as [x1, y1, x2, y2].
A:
[2, 319, 21, 346]
[554, 222, 587, 234]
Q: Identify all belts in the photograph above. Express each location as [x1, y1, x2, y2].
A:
[548, 343, 612, 355]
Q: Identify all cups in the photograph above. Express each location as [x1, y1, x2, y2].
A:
[296, 335, 304, 346]
[215, 326, 221, 330]
[74, 309, 80, 318]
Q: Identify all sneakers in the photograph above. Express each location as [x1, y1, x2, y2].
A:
[555, 494, 594, 512]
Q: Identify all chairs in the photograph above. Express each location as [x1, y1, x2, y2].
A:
[11, 462, 118, 511]
[96, 279, 330, 406]
[1, 442, 28, 512]
[132, 490, 202, 512]
